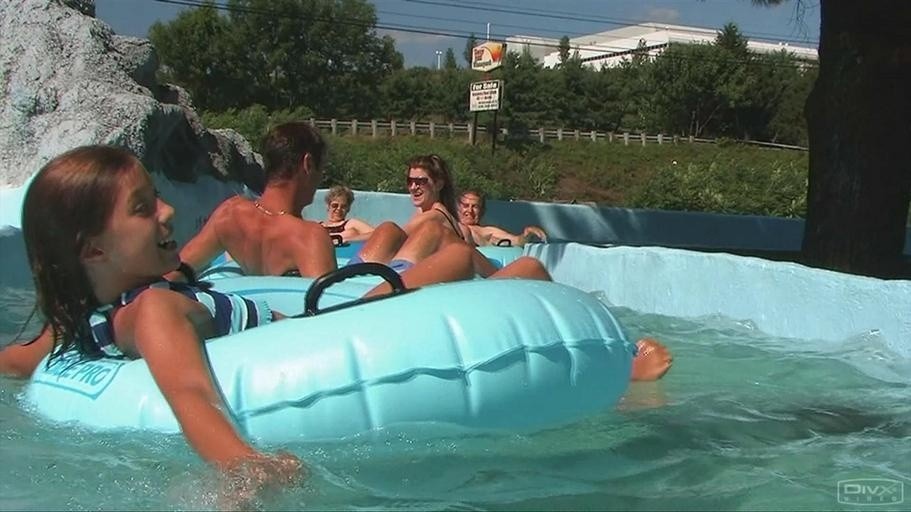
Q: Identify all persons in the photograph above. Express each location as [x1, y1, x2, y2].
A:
[161, 122, 470, 297]
[314, 186, 376, 244]
[0, 146, 676, 512]
[397, 152, 464, 240]
[457, 187, 550, 251]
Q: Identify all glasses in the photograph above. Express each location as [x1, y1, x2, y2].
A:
[408, 176, 429, 185]
[329, 203, 349, 209]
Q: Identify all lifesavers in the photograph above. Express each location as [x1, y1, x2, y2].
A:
[191, 256, 416, 289]
[329, 234, 368, 259]
[473, 238, 525, 272]
[29, 260, 641, 448]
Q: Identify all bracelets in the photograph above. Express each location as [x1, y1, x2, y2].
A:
[256, 202, 299, 218]
